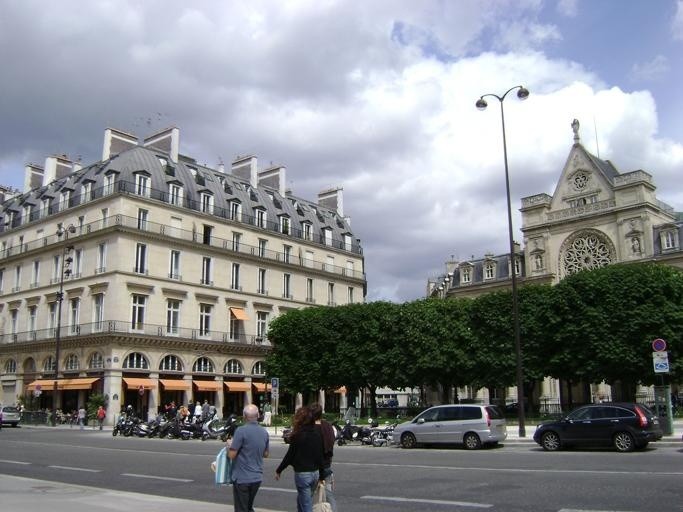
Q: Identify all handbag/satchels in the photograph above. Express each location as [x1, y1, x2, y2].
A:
[312, 503, 331, 512]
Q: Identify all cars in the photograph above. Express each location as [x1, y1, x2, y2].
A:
[532, 400, 668, 454]
[382, 398, 400, 407]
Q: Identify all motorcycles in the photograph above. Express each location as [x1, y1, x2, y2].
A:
[109, 409, 236, 442]
[280, 414, 403, 448]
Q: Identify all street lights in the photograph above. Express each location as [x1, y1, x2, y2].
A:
[475, 83, 532, 439]
[50, 223, 79, 428]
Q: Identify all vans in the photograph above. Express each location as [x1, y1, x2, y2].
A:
[0, 404, 22, 428]
[391, 402, 512, 450]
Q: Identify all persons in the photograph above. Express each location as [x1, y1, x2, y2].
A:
[164, 400, 216, 426]
[16, 403, 22, 412]
[310, 403, 337, 512]
[632, 238, 639, 253]
[263, 402, 272, 427]
[535, 255, 541, 267]
[273, 407, 326, 512]
[97, 406, 106, 430]
[595, 390, 608, 405]
[77, 406, 87, 431]
[226, 403, 269, 512]
